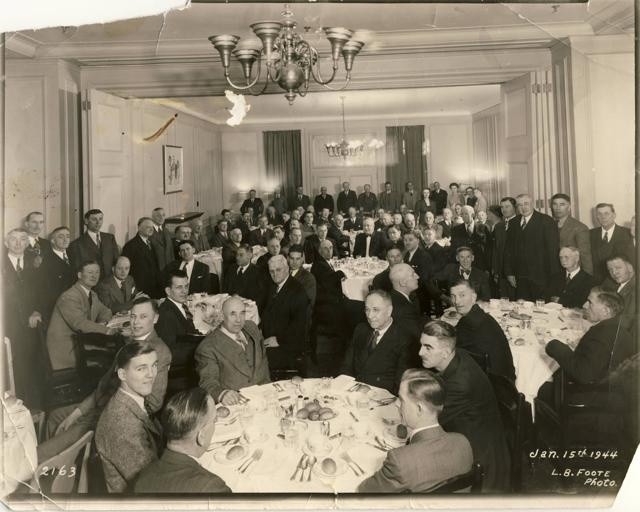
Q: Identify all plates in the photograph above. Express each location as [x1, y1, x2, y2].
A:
[207, 380, 409, 477]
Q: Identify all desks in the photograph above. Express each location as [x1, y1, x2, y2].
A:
[164, 212, 205, 235]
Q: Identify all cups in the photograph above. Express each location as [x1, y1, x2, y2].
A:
[331, 255, 378, 278]
[187, 294, 239, 322]
[481, 295, 581, 342]
[212, 246, 223, 257]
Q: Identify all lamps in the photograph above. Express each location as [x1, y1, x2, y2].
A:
[324, 95, 363, 161]
[208, 3, 365, 106]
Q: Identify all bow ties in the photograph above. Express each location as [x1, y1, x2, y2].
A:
[461, 269, 471, 275]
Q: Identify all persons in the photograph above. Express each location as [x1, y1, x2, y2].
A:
[419, 318, 509, 494]
[4, 181, 637, 411]
[134, 387, 231, 494]
[356, 366, 473, 494]
[36, 298, 172, 467]
[93, 340, 160, 494]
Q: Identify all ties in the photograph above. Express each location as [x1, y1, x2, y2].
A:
[144, 398, 155, 422]
[182, 303, 195, 330]
[16, 258, 23, 284]
[602, 232, 608, 245]
[521, 216, 527, 230]
[33, 239, 39, 254]
[146, 239, 151, 249]
[564, 273, 571, 288]
[121, 281, 126, 302]
[237, 267, 244, 278]
[88, 291, 92, 306]
[63, 252, 68, 263]
[97, 234, 100, 248]
[182, 263, 187, 278]
[367, 331, 379, 356]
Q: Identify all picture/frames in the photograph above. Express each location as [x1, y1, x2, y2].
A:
[162, 144, 184, 195]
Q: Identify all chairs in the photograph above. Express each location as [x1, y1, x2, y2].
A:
[29, 319, 126, 493]
[403, 346, 525, 493]
[271, 313, 318, 378]
[535, 369, 632, 488]
